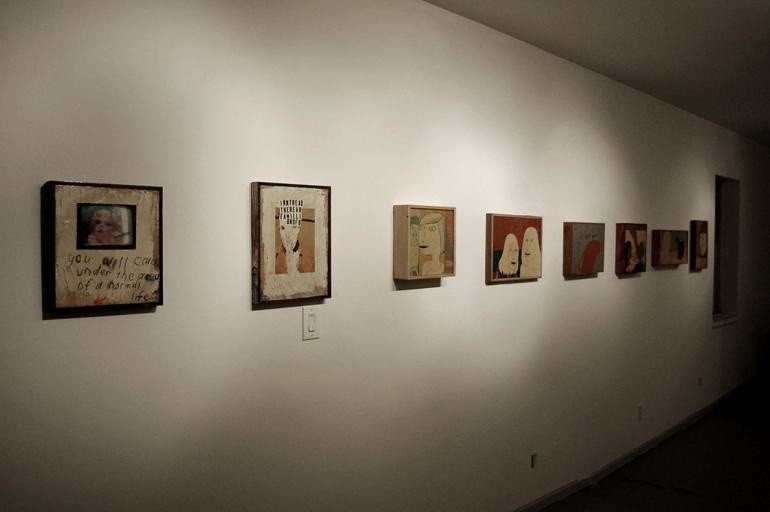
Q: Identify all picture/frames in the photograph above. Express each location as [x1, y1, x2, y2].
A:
[488, 213, 544, 281]
[394, 203, 457, 281]
[562, 220, 707, 278]
[251, 182, 332, 304]
[41, 179, 164, 312]
